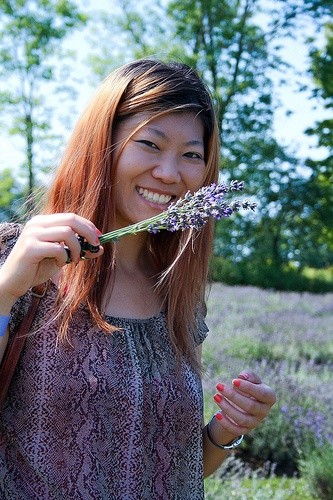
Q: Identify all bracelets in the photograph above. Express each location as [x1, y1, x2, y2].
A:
[0, 314, 12, 336]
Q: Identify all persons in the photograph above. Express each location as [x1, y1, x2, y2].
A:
[1, 53, 279, 498]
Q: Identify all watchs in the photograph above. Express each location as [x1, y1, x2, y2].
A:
[206, 411, 247, 451]
